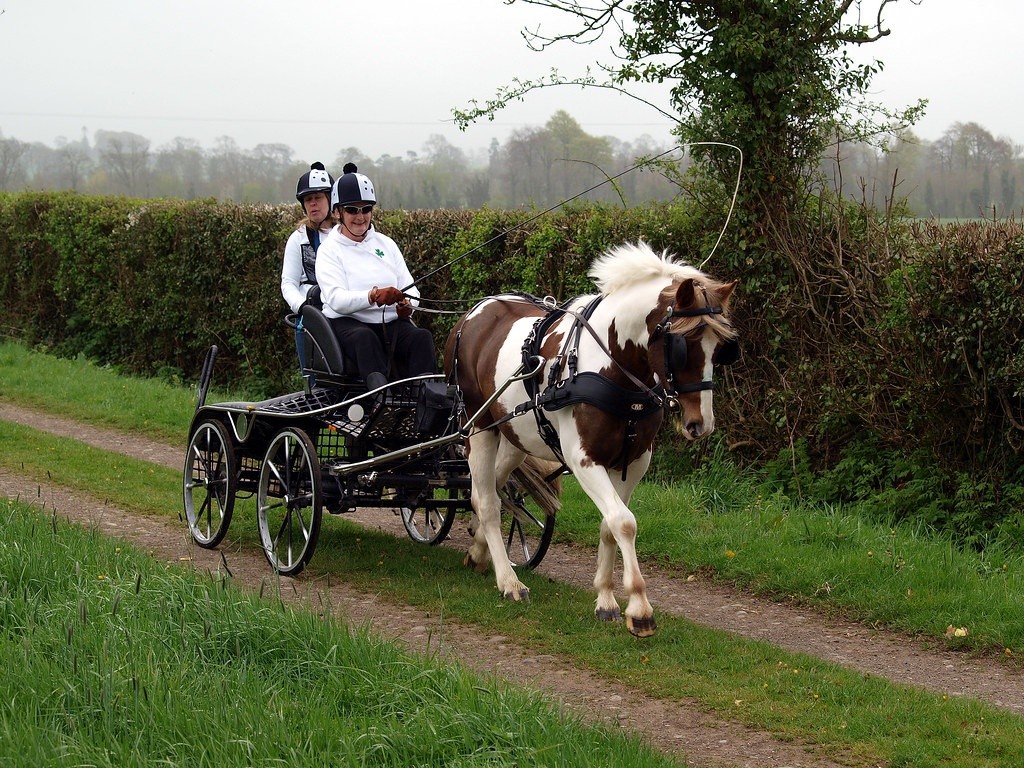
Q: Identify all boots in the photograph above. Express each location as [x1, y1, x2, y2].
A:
[367, 372, 392, 414]
[413, 372, 434, 401]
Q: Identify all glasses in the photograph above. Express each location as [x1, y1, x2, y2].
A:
[342, 204, 372, 215]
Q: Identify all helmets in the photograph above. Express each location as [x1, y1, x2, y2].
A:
[331, 163, 376, 213]
[296, 161, 335, 203]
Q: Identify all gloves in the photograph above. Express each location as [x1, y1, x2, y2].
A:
[370, 287, 403, 307]
[396, 300, 412, 317]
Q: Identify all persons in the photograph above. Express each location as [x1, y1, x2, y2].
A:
[280, 161, 336, 314]
[315, 163, 437, 410]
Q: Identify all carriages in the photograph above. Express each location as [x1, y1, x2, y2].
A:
[180, 239, 740, 638]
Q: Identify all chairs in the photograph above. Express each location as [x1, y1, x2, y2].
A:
[297, 285, 357, 384]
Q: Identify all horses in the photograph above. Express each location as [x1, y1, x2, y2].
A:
[440, 235, 744, 638]
